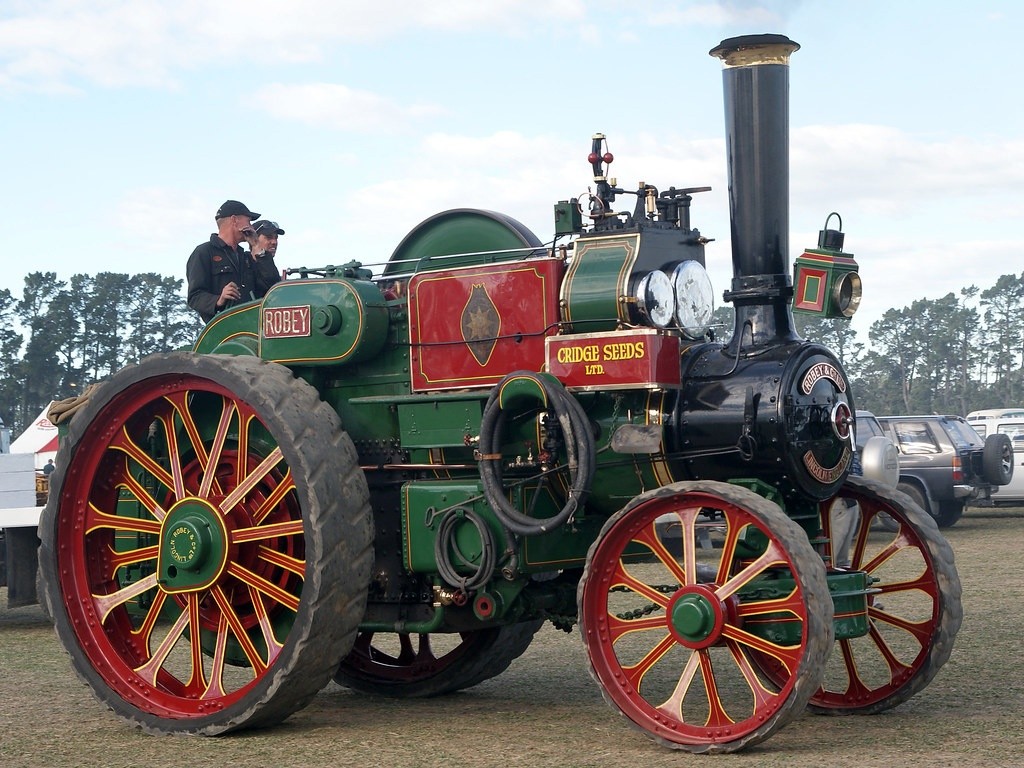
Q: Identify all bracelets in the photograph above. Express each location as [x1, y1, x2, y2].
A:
[257, 249, 266, 260]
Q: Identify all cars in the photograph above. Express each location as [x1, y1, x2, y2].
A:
[966, 418, 1024, 507]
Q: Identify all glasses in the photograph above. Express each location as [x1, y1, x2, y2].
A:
[255, 222, 279, 231]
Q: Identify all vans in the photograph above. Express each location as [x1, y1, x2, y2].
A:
[965, 409, 1024, 422]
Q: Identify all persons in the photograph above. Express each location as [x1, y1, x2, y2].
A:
[186, 199, 280, 325]
[44, 459, 55, 474]
[249, 220, 285, 259]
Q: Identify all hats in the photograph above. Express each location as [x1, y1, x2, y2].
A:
[251, 220, 284, 235]
[215, 200, 261, 221]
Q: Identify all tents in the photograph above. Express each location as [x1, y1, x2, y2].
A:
[7, 398, 59, 475]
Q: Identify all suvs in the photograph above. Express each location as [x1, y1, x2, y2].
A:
[853, 410, 901, 539]
[875, 414, 1014, 534]
